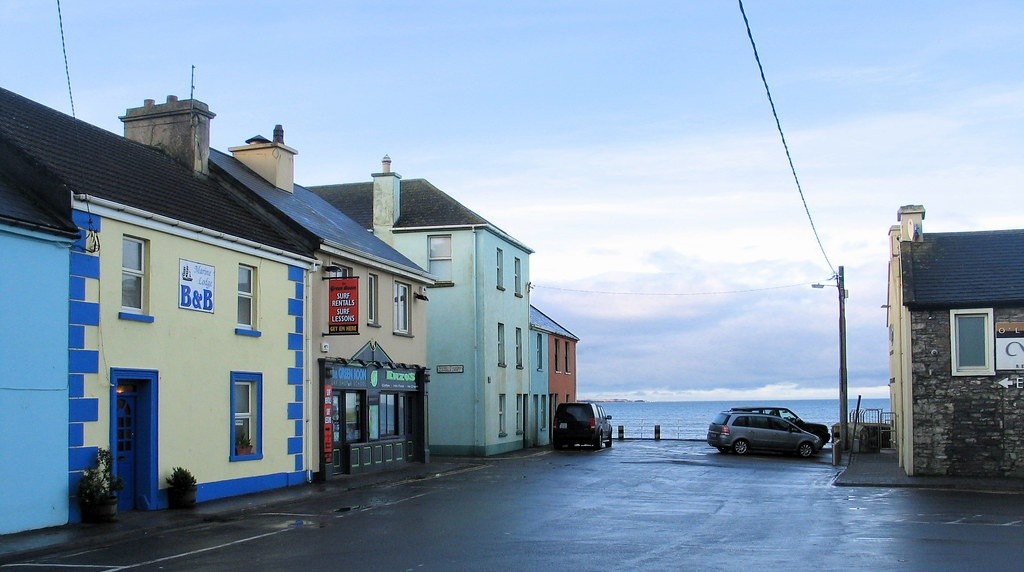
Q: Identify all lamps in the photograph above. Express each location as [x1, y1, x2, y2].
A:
[414, 292, 429, 301]
[324, 265, 341, 273]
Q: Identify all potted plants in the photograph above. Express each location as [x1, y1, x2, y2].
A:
[166, 467, 197, 506]
[78, 448, 122, 522]
[235, 430, 253, 455]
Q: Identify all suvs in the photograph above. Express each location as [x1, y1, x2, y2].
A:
[706, 410, 822, 458]
[730, 406, 831, 447]
[552, 402, 613, 450]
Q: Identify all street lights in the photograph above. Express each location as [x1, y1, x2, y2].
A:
[811, 265, 848, 451]
